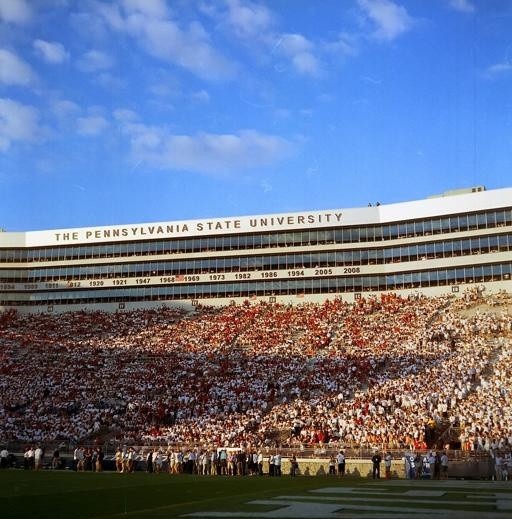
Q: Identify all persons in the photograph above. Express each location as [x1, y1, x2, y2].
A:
[0, 284, 511, 479]
